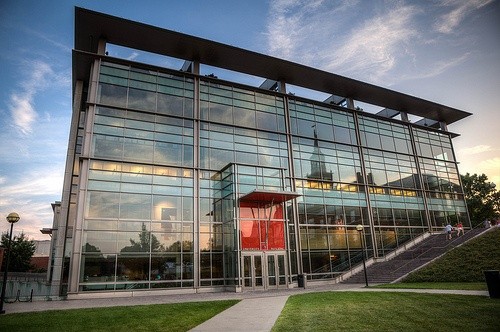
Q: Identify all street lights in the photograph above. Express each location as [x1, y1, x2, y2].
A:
[0, 212, 20, 313]
[356, 223, 369, 286]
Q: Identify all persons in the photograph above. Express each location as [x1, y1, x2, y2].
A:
[456, 222, 466, 237]
[484, 217, 489, 229]
[442, 222, 453, 241]
